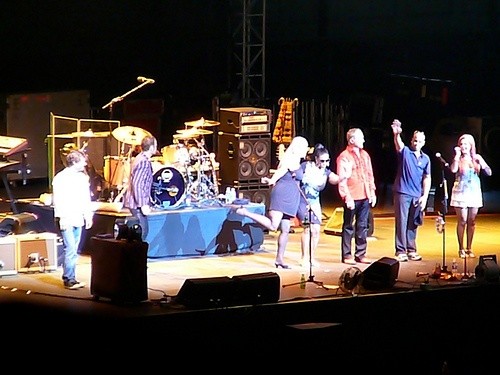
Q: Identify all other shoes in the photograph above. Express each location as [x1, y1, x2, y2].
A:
[64, 284, 79, 289]
[458, 250, 467, 258]
[301, 258, 318, 267]
[359, 257, 372, 264]
[75, 281, 84, 286]
[466, 248, 475, 257]
[344, 258, 357, 264]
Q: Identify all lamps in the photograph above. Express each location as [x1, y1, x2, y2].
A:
[475, 255, 500, 296]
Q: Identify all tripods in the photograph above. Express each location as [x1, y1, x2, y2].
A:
[278, 177, 329, 292]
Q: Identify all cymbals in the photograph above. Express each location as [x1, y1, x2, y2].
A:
[173, 133, 199, 138]
[184, 120, 220, 126]
[176, 129, 214, 134]
[112, 126, 153, 145]
[72, 131, 109, 137]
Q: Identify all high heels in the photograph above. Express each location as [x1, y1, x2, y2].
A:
[275, 263, 290, 269]
[230, 206, 243, 215]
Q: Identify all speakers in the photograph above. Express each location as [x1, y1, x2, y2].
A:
[90, 237, 149, 302]
[17, 236, 56, 271]
[232, 271, 281, 305]
[176, 276, 232, 310]
[217, 132, 271, 232]
[324, 207, 374, 236]
[358, 256, 400, 291]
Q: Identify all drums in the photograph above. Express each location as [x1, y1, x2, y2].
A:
[161, 143, 191, 168]
[150, 161, 187, 210]
[103, 155, 129, 190]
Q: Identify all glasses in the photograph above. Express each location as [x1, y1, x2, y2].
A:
[317, 158, 330, 162]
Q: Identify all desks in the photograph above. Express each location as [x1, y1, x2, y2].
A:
[140, 200, 265, 256]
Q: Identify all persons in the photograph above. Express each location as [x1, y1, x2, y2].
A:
[125, 136, 157, 241]
[269, 144, 351, 266]
[235, 136, 309, 269]
[450, 134, 492, 258]
[187, 152, 226, 201]
[390, 119, 431, 261]
[336, 128, 376, 265]
[52, 152, 94, 289]
[132, 146, 139, 157]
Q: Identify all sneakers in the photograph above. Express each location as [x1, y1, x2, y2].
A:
[407, 252, 422, 261]
[395, 253, 408, 262]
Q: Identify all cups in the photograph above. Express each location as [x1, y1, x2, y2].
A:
[163, 201, 170, 209]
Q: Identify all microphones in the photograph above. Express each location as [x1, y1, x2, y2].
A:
[137, 76, 155, 83]
[436, 153, 448, 166]
[40, 257, 48, 262]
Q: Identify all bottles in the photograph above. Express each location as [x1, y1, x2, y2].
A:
[452, 259, 458, 275]
[300, 274, 305, 289]
[226, 187, 232, 204]
[231, 188, 235, 202]
